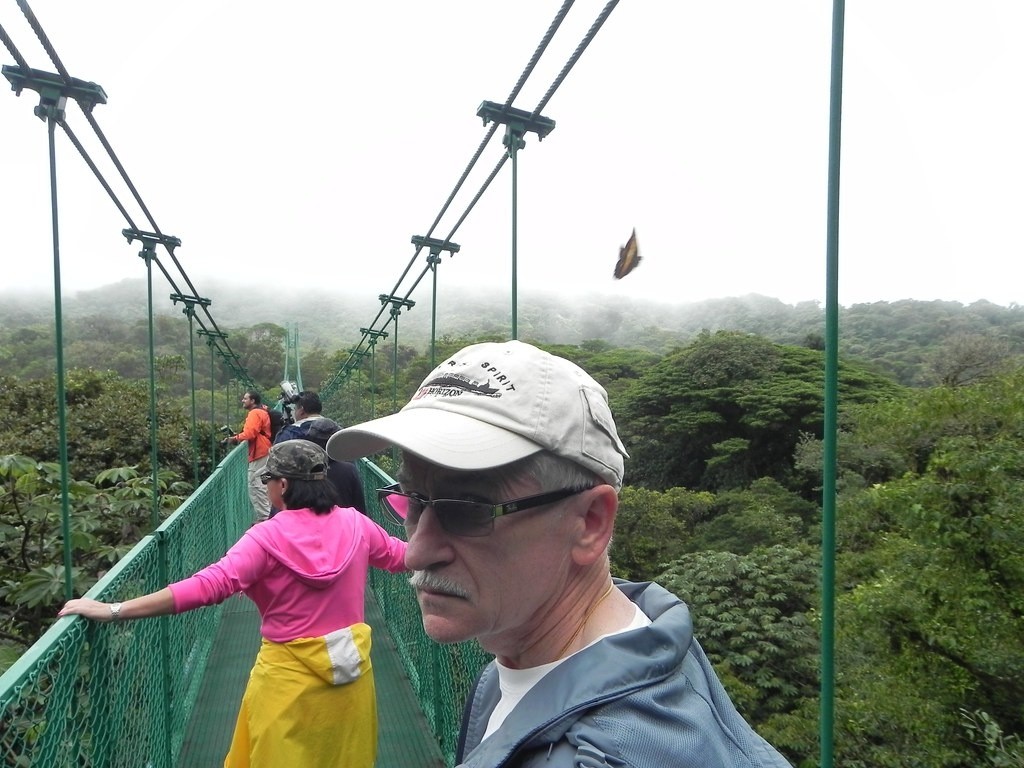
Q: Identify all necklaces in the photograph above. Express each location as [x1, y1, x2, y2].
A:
[554, 579, 613, 661]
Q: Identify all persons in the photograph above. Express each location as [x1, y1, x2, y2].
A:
[221, 391, 272, 527]
[263, 391, 366, 518]
[59, 439, 408, 768]
[327, 340, 796, 768]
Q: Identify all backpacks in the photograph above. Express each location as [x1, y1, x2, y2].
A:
[250, 407, 285, 443]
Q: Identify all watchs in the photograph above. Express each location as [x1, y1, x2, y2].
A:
[110, 603, 121, 619]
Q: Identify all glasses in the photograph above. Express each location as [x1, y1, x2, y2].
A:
[260, 472, 280, 484]
[375, 481, 594, 537]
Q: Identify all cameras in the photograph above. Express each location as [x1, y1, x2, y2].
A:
[220, 426, 241, 447]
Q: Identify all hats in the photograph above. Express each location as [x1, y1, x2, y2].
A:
[327, 340, 629, 503]
[267, 438, 328, 480]
